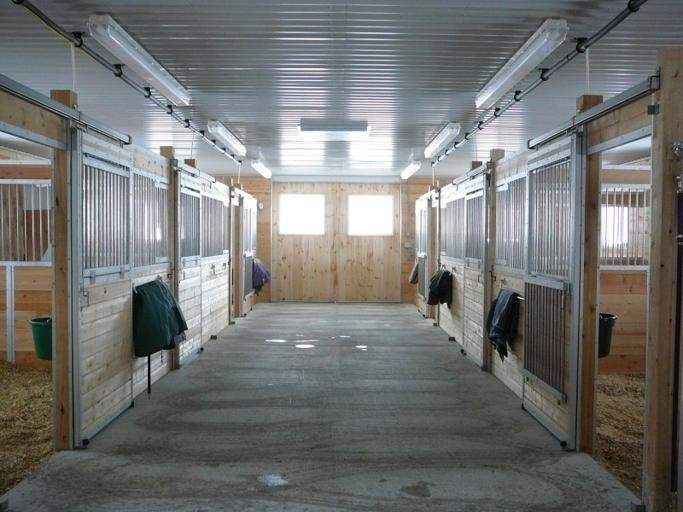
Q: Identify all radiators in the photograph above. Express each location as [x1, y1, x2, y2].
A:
[244, 253, 256, 301]
[522, 276, 569, 406]
[416, 254, 426, 302]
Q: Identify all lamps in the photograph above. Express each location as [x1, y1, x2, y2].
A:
[401, 161, 421, 179]
[251, 161, 271, 179]
[424, 123, 460, 159]
[88, 13, 190, 107]
[207, 120, 246, 158]
[474, 19, 568, 111]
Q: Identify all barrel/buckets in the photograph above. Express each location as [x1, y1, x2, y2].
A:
[29, 317, 52, 360]
[598, 313, 618, 359]
